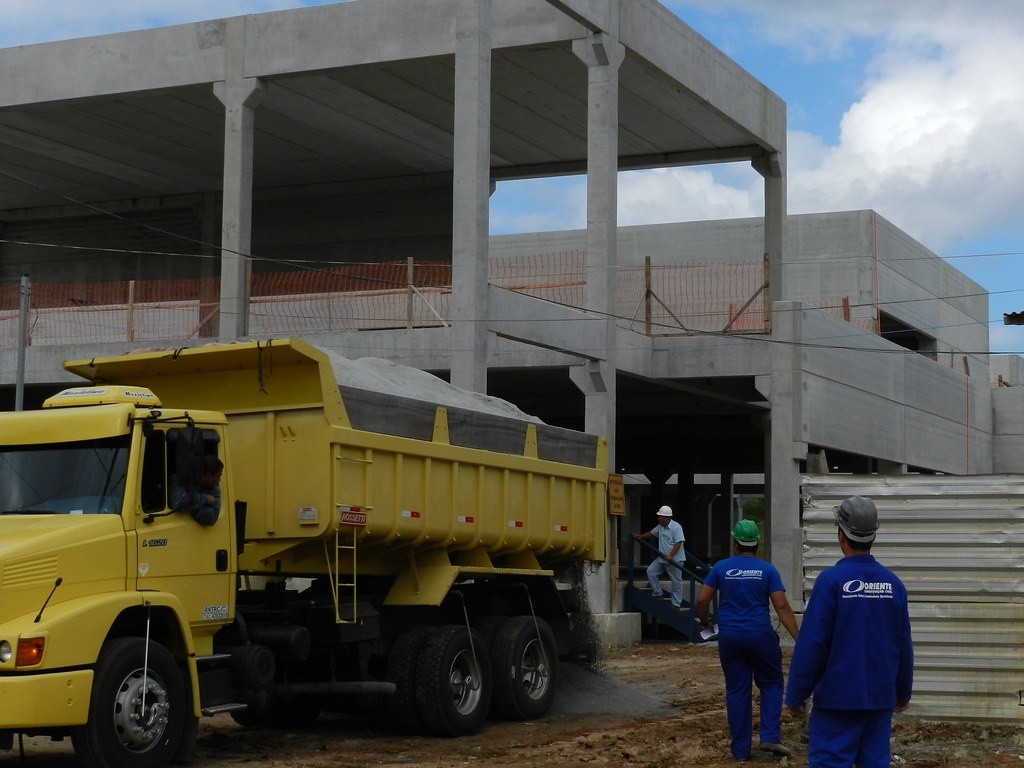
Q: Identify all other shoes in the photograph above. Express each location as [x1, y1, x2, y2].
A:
[732, 754, 752, 764]
[760, 742, 791, 756]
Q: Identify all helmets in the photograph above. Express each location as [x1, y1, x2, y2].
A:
[656, 506, 673, 516]
[832, 495, 880, 543]
[731, 519, 763, 546]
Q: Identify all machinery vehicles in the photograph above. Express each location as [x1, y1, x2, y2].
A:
[0, 338, 609, 768]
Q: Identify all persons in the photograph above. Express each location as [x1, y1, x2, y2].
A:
[696, 518, 800, 761]
[784, 496, 914, 768]
[632, 504, 686, 608]
[169, 454, 223, 526]
